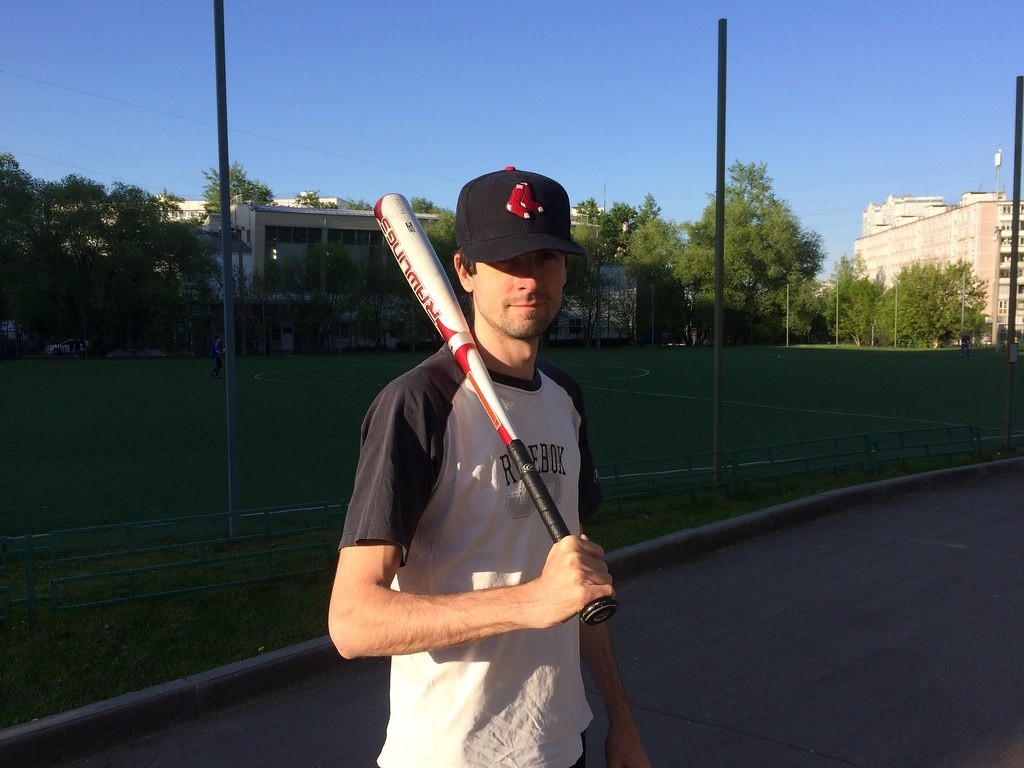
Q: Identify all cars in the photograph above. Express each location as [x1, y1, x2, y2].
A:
[42, 339, 93, 357]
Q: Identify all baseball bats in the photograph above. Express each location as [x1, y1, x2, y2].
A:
[373, 193, 619, 628]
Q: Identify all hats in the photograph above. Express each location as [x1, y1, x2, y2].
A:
[455, 166, 583, 263]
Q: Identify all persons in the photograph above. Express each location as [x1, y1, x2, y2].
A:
[959, 331, 972, 356]
[326, 165, 653, 768]
[208, 336, 226, 379]
